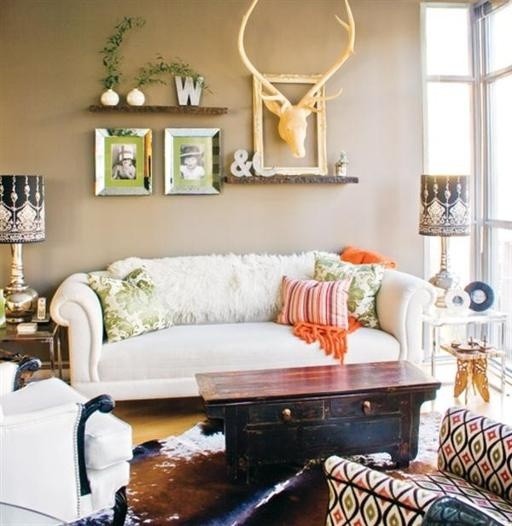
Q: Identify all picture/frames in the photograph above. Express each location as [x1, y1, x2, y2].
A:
[93, 127, 153, 197]
[248, 71, 329, 179]
[162, 127, 223, 196]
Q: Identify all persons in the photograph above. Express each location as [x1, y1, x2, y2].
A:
[112, 145, 137, 180]
[179, 147, 206, 180]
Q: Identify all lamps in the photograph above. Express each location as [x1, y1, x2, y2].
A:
[0, 172, 46, 324]
[419, 171, 472, 290]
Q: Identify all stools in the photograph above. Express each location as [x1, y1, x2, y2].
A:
[441, 342, 504, 403]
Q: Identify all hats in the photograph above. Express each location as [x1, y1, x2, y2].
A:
[179, 146, 203, 158]
[117, 144, 133, 163]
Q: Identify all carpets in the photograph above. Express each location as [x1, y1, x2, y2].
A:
[83, 408, 442, 525]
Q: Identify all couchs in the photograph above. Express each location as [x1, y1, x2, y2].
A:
[0, 350, 133, 525]
[50, 252, 433, 402]
[324, 406, 512, 526]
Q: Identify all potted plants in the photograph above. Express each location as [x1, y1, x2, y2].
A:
[124, 54, 210, 104]
[93, 13, 146, 105]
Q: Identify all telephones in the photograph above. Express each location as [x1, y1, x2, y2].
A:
[32, 297, 51, 324]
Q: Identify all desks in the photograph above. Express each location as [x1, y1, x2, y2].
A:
[425, 311, 507, 394]
[1, 315, 62, 379]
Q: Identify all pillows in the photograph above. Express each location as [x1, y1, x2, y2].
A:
[83, 271, 175, 342]
[314, 252, 384, 331]
[275, 275, 351, 330]
